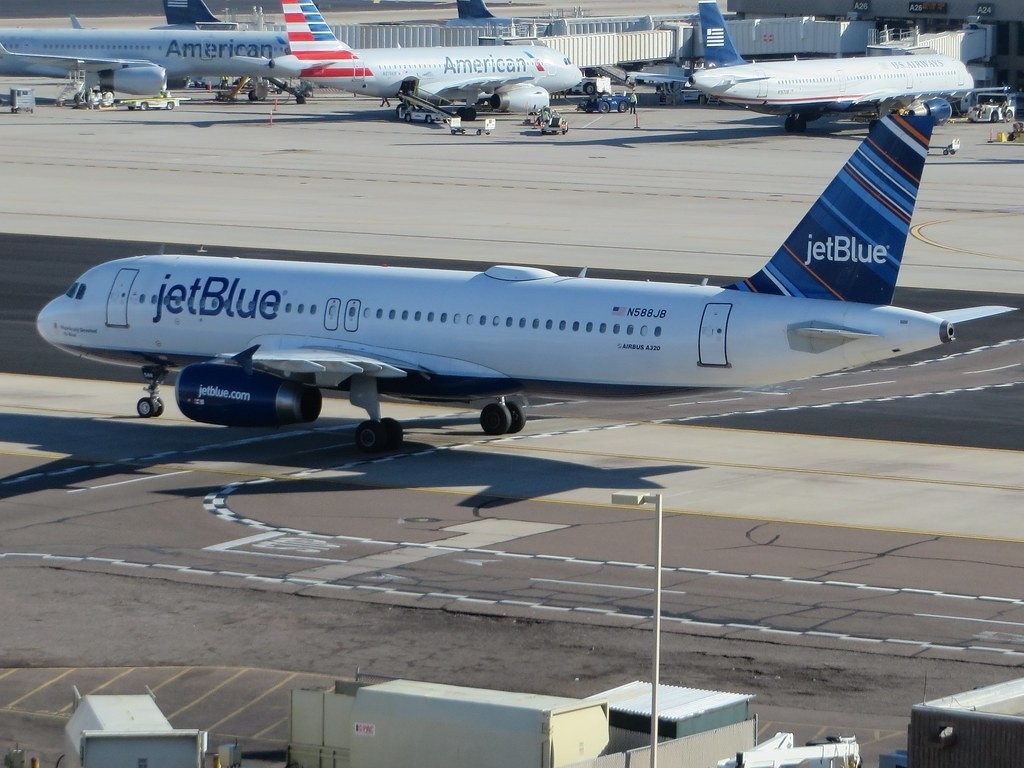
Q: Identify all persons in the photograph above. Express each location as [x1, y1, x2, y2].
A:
[542, 106, 550, 123]
[1001, 100, 1009, 124]
[630, 91, 637, 114]
[380, 97, 390, 107]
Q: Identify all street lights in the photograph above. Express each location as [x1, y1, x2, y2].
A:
[612, 493, 662, 768]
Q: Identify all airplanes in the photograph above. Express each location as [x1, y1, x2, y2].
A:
[38, 113, 1021, 446]
[0, 15, 289, 108]
[231, 0, 582, 122]
[689, 1, 974, 130]
